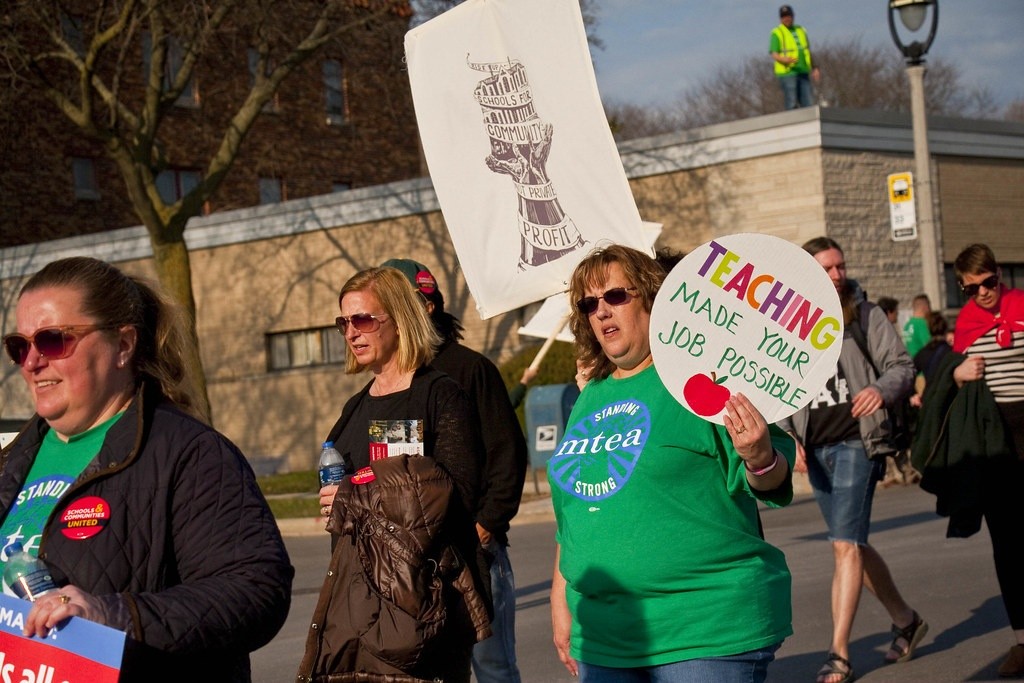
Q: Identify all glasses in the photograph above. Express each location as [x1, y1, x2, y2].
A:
[575, 286, 637, 314]
[2, 322, 139, 368]
[960, 273, 998, 297]
[335, 313, 391, 336]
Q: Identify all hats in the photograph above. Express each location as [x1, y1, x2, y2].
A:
[379, 259, 443, 302]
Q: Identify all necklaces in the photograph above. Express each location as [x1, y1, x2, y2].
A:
[375, 372, 404, 396]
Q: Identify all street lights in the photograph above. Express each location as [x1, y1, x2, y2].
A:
[887, 0, 948, 318]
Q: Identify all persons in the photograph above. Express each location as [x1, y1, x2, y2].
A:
[769, 5, 815, 109]
[875, 295, 954, 485]
[0, 256, 297, 683]
[317, 266, 480, 683]
[380, 258, 528, 683]
[952, 244, 1024, 676]
[774, 237, 928, 683]
[546, 246, 796, 683]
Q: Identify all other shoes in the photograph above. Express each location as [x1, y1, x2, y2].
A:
[998, 646, 1024, 676]
[901, 464, 921, 485]
[878, 457, 904, 488]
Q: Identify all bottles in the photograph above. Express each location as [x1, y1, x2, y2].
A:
[3, 542, 55, 604]
[319, 441, 347, 508]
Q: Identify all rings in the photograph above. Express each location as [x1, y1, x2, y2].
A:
[324, 506, 328, 513]
[736, 425, 746, 434]
[61, 596, 67, 603]
[581, 370, 586, 377]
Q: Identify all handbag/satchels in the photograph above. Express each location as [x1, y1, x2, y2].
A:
[886, 397, 919, 452]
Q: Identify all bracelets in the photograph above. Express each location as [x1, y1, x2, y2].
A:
[744, 447, 778, 476]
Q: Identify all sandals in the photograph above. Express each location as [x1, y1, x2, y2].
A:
[816, 653, 854, 683]
[884, 609, 928, 663]
